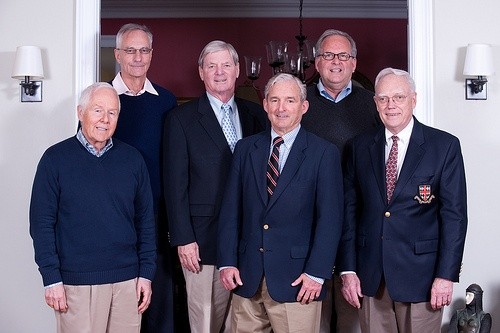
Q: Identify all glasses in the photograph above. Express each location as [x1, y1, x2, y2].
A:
[118, 48, 151, 54]
[317, 52, 354, 61]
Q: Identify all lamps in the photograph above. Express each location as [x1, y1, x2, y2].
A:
[10, 45, 45, 102]
[244, 0, 317, 92]
[461, 41, 494, 100]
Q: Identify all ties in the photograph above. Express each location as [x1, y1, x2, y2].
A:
[385, 136, 399, 204]
[266, 137, 284, 200]
[221, 104, 238, 154]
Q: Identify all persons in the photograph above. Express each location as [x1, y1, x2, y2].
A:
[163, 39, 271, 333]
[77, 23, 177, 333]
[216, 73, 344, 333]
[301, 29, 382, 333]
[338, 68, 467, 333]
[29, 82, 157, 333]
